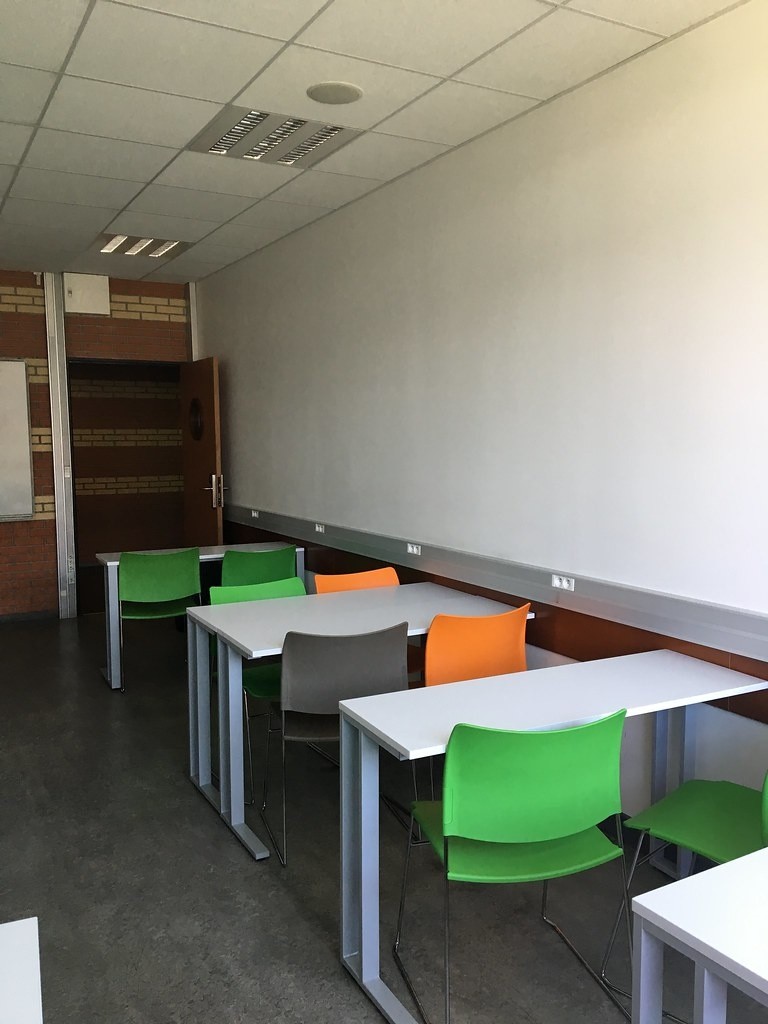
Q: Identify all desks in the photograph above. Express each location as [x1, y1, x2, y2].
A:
[336, 649, 768, 1024]
[181, 581, 479, 814]
[630, 846, 768, 1024]
[217, 597, 536, 862]
[92, 542, 306, 691]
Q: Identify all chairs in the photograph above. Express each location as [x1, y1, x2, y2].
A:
[209, 576, 307, 803]
[392, 709, 635, 1024]
[377, 602, 532, 849]
[258, 618, 422, 868]
[310, 565, 402, 774]
[222, 544, 297, 587]
[601, 769, 768, 1024]
[117, 547, 203, 694]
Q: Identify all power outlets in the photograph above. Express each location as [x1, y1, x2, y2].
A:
[315, 524, 325, 534]
[407, 542, 422, 556]
[551, 574, 575, 592]
[251, 510, 260, 518]
